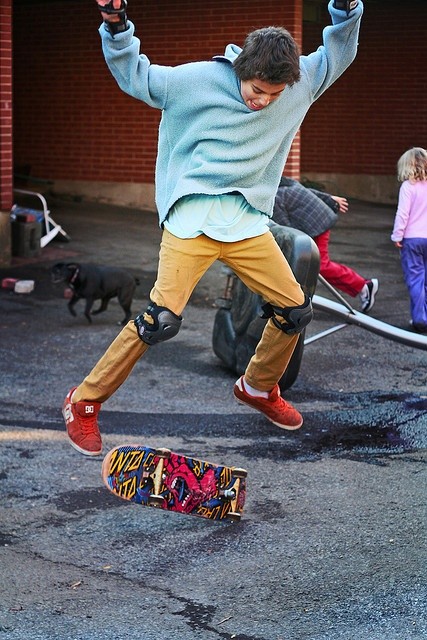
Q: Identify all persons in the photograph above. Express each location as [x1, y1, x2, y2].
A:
[390, 148, 427, 334]
[61, 0, 363, 456]
[270, 176, 379, 313]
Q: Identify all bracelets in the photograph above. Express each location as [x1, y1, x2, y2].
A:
[333, 0, 359, 17]
[103, 13, 129, 40]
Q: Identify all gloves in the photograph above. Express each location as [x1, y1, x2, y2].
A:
[99, 0, 130, 40]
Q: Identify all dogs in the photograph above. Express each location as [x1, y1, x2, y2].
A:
[50, 262, 140, 325]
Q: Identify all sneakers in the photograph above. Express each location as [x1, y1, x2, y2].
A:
[62, 387, 104, 456]
[233, 375, 303, 431]
[360, 278, 379, 312]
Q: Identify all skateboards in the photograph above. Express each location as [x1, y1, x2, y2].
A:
[100, 444, 248, 524]
